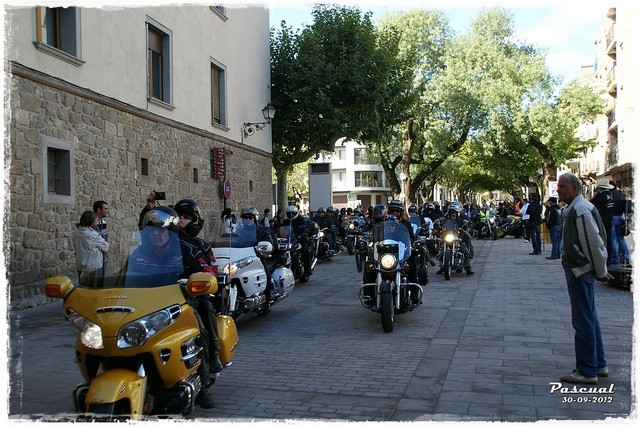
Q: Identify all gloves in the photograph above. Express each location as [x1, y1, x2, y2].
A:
[301, 232, 307, 239]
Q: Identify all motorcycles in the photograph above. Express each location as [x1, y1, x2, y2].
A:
[341, 216, 365, 255]
[432, 215, 471, 280]
[353, 221, 369, 273]
[205, 217, 295, 320]
[310, 221, 335, 261]
[45, 226, 239, 422]
[276, 221, 320, 282]
[495, 215, 527, 239]
[469, 210, 497, 240]
[410, 213, 430, 286]
[355, 220, 425, 332]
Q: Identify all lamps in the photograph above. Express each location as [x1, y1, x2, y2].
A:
[247, 101, 276, 126]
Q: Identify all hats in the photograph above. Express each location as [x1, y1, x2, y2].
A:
[596, 178, 615, 189]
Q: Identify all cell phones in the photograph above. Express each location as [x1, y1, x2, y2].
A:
[97, 224, 106, 229]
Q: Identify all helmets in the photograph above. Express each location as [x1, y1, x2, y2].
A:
[453, 202, 463, 208]
[388, 200, 404, 216]
[425, 204, 436, 213]
[433, 201, 440, 207]
[318, 207, 327, 214]
[326, 206, 334, 213]
[340, 208, 347, 212]
[286, 205, 299, 220]
[354, 208, 360, 213]
[347, 208, 352, 211]
[368, 206, 374, 212]
[334, 208, 339, 214]
[408, 205, 418, 215]
[174, 199, 204, 237]
[374, 204, 386, 220]
[447, 205, 461, 218]
[139, 207, 180, 239]
[240, 207, 259, 221]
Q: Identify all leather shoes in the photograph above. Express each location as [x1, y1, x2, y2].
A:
[560, 372, 598, 384]
[572, 364, 609, 377]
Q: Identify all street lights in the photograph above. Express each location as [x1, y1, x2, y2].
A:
[537, 163, 545, 252]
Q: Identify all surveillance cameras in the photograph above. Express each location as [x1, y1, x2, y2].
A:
[245, 125, 255, 137]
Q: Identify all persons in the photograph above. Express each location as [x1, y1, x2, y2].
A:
[315, 207, 333, 256]
[274, 209, 283, 225]
[334, 203, 389, 234]
[363, 199, 419, 304]
[333, 208, 341, 250]
[587, 179, 611, 264]
[120, 206, 215, 409]
[327, 207, 336, 254]
[439, 203, 475, 275]
[366, 206, 373, 218]
[360, 204, 385, 232]
[420, 199, 447, 229]
[546, 197, 561, 258]
[233, 207, 277, 315]
[221, 207, 237, 226]
[556, 172, 615, 384]
[368, 213, 419, 252]
[525, 195, 543, 256]
[521, 196, 531, 242]
[279, 205, 313, 282]
[608, 181, 630, 262]
[558, 198, 568, 218]
[408, 206, 436, 267]
[258, 208, 271, 228]
[541, 201, 550, 225]
[463, 199, 513, 243]
[91, 200, 109, 286]
[72, 209, 109, 288]
[137, 191, 223, 375]
[514, 194, 522, 216]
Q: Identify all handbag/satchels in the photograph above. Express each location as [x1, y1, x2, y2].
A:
[616, 219, 631, 237]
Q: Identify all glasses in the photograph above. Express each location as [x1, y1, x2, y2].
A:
[390, 207, 401, 213]
[178, 212, 193, 219]
[244, 216, 255, 220]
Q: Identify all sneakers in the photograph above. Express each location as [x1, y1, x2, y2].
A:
[363, 299, 374, 305]
[545, 255, 556, 259]
[529, 251, 540, 255]
[210, 351, 224, 374]
[307, 266, 312, 275]
[437, 268, 443, 274]
[467, 270, 474, 275]
[196, 385, 215, 409]
[410, 296, 423, 305]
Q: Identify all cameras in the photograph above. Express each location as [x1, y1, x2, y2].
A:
[153, 191, 166, 199]
